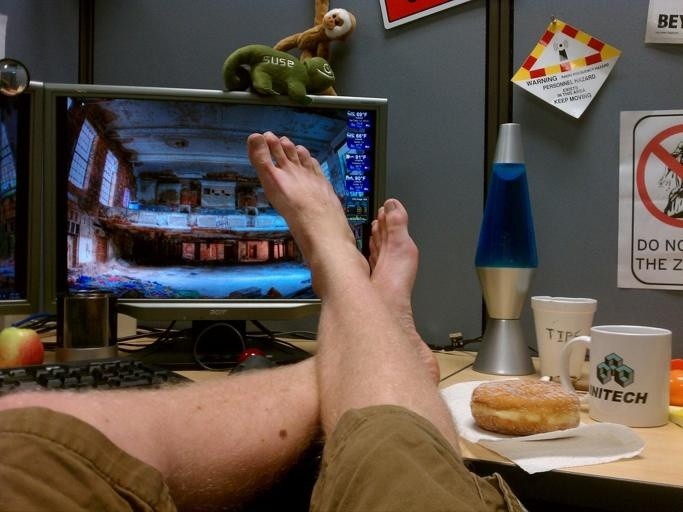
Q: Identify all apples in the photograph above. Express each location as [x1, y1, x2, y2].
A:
[0, 326, 45, 369]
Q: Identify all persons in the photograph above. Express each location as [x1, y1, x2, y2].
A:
[0, 129, 534, 512]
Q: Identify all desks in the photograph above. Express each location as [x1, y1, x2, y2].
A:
[0, 329, 683, 512]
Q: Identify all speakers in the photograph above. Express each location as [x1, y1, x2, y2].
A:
[55, 291, 118, 364]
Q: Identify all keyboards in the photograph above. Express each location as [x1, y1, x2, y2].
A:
[0, 355, 197, 402]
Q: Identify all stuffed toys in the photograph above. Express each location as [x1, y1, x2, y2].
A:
[221, 42, 337, 104]
[273, 8, 356, 98]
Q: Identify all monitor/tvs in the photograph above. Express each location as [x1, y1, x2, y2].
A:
[0, 80, 44, 335]
[44, 81, 390, 371]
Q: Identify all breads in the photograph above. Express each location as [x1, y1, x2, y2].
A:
[470, 379, 583, 436]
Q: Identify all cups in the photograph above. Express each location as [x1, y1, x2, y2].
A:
[531, 296, 672, 428]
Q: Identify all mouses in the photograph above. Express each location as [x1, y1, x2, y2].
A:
[226, 353, 277, 376]
